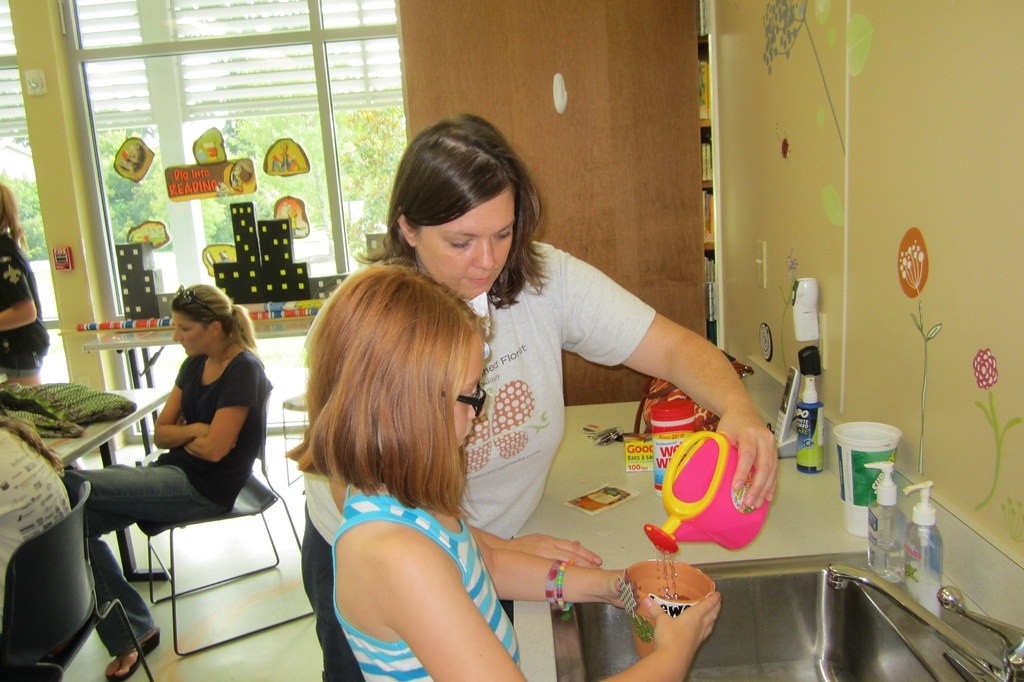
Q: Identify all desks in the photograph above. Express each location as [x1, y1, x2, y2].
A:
[81, 320, 318, 452]
[497, 401, 881, 682]
[34, 387, 177, 580]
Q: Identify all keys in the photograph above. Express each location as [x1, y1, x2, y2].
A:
[593, 427, 620, 445]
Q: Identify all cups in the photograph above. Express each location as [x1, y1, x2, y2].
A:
[833, 421, 902, 538]
[627, 561, 715, 658]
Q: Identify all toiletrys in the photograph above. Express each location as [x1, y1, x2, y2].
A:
[902, 480, 944, 618]
[863, 460, 911, 584]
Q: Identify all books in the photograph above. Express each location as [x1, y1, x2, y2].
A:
[702, 143, 712, 181]
[698, 60, 712, 119]
[705, 257, 717, 321]
[703, 191, 714, 242]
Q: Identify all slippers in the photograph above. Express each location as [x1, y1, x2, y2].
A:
[105, 627, 162, 682]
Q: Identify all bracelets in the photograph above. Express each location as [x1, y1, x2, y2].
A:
[556, 562, 576, 624]
[545, 560, 562, 605]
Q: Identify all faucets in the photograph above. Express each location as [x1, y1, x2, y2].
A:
[824, 563, 1024, 682]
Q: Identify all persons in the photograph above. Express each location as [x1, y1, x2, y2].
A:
[0, 182, 50, 385]
[60, 284, 272, 682]
[0, 402, 71, 635]
[299, 114, 779, 682]
[286, 264, 720, 682]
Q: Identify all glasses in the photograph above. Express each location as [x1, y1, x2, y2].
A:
[173, 284, 219, 319]
[440, 379, 489, 418]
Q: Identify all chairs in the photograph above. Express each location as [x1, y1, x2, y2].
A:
[0, 480, 156, 681]
[136, 392, 317, 656]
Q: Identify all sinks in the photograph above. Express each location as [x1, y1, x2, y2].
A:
[550, 556, 945, 682]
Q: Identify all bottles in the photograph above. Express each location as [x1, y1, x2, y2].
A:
[651, 400, 694, 497]
[795, 376, 824, 474]
